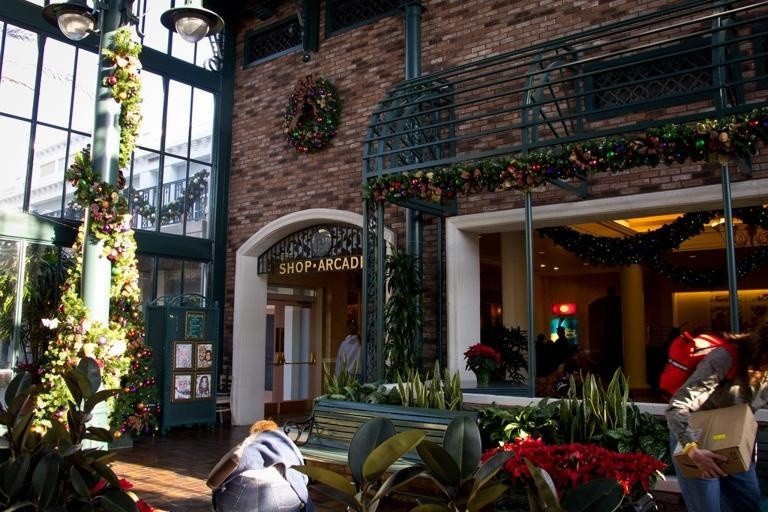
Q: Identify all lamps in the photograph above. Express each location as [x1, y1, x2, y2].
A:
[43, 1, 101, 42]
[159, 1, 226, 45]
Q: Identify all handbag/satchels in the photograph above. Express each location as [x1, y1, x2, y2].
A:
[207, 445, 243, 489]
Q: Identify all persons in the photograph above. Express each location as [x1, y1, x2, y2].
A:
[199, 376, 210, 397]
[202, 350, 211, 366]
[334, 327, 362, 384]
[536, 327, 576, 376]
[210, 420, 309, 512]
[663, 317, 767, 511]
[175, 381, 190, 399]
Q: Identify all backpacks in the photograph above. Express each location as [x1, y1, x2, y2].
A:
[658, 331, 738, 396]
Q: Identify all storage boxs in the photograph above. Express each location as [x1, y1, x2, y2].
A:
[672, 401, 758, 479]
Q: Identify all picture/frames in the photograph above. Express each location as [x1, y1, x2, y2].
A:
[168, 310, 216, 404]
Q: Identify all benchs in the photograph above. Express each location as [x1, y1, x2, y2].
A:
[281, 399, 482, 494]
